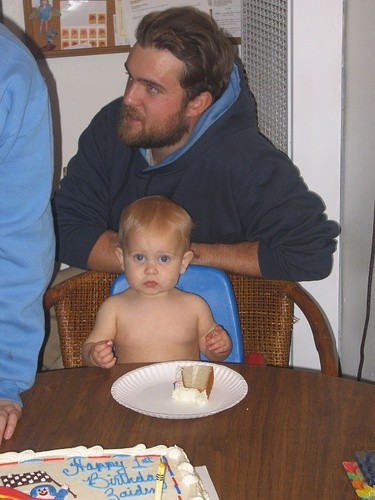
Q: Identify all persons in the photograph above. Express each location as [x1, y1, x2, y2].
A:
[0, 27, 59, 447]
[51, 5, 341, 281]
[81, 196, 232, 368]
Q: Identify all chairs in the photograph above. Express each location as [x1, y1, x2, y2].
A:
[110, 265, 243, 364]
[45, 249, 339, 377]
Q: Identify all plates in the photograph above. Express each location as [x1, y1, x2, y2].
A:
[110, 360, 249, 419]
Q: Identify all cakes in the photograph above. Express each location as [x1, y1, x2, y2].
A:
[171, 364, 213, 405]
[0, 442, 210, 500]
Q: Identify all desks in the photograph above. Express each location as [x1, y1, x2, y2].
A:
[0, 360, 375, 500]
